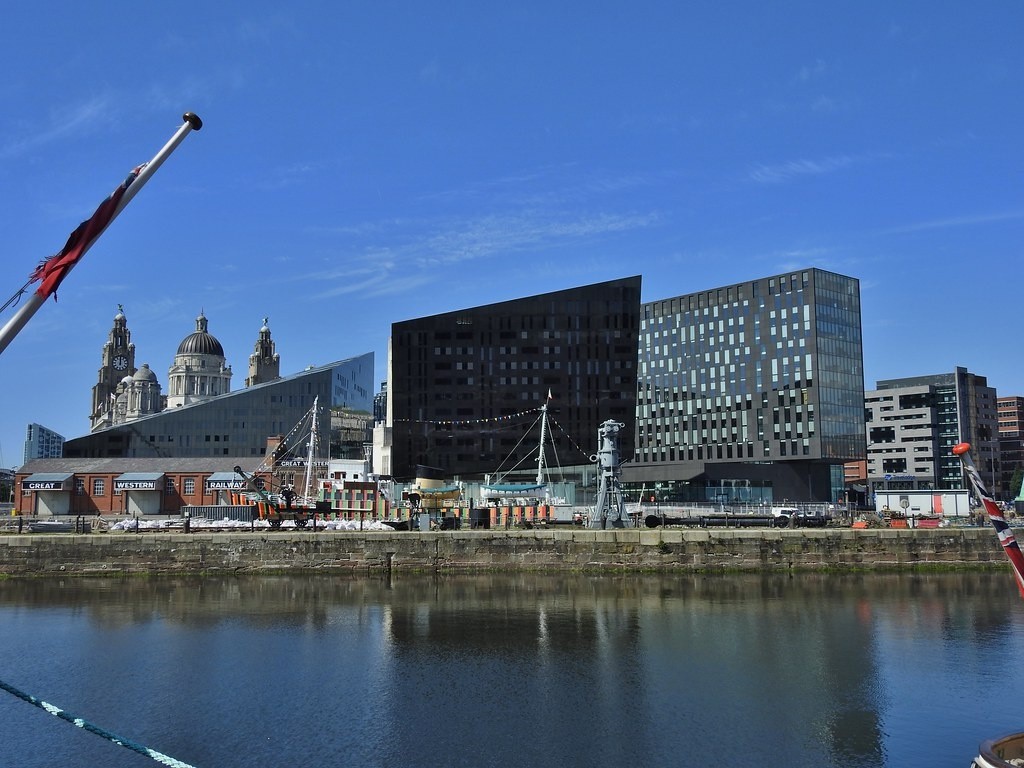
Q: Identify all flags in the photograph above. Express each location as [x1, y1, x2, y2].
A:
[964, 466, 1024, 600]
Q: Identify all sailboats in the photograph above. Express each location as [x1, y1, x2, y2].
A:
[226, 395, 331, 521]
[479, 404, 573, 524]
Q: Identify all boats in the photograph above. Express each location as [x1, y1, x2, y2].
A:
[969, 729, 1024, 768]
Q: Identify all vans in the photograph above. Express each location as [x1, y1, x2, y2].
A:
[771, 507, 801, 518]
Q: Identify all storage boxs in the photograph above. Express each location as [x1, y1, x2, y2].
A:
[420, 499, 469, 508]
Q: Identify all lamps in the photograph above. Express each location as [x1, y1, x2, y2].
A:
[17, 481, 21, 485]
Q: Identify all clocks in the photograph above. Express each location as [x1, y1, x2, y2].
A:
[112, 355, 129, 371]
